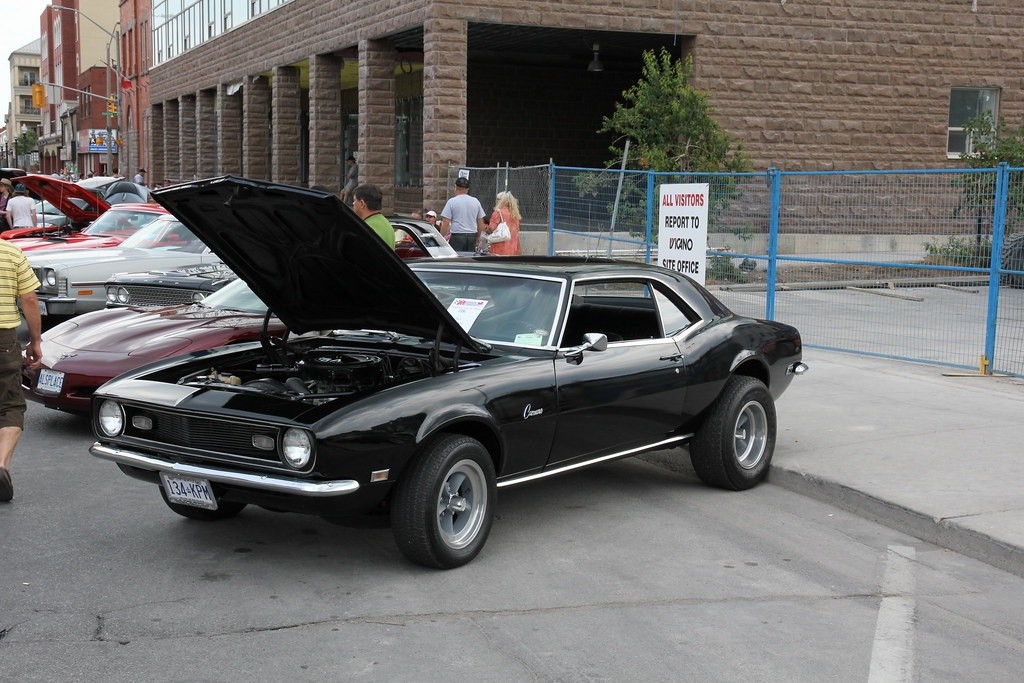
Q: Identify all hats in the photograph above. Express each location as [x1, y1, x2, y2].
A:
[1, 178, 14, 190]
[139, 169, 148, 173]
[15, 184, 26, 192]
[425, 211, 437, 218]
[456, 177, 469, 188]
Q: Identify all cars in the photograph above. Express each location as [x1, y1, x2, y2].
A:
[103, 214, 500, 311]
[89, 173, 809, 568]
[0, 174, 196, 253]
[17, 214, 223, 330]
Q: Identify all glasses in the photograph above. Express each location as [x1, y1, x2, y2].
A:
[426, 216, 434, 218]
[496, 199, 501, 201]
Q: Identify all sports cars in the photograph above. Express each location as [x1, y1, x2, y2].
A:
[19, 266, 496, 417]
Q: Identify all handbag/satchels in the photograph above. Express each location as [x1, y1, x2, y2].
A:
[488, 209, 512, 243]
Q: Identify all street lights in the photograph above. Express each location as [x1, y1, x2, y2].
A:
[51, 5, 126, 176]
[4, 135, 9, 168]
[106, 20, 120, 176]
[13, 131, 19, 168]
[22, 124, 27, 170]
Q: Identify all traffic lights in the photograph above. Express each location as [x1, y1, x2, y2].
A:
[115, 140, 120, 146]
[108, 96, 115, 113]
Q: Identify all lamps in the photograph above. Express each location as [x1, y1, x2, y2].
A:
[586, 43, 604, 71]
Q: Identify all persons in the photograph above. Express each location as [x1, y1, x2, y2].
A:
[485, 190, 522, 256]
[53, 165, 110, 184]
[353, 183, 397, 254]
[6, 183, 39, 230]
[0, 238, 43, 503]
[0, 178, 15, 235]
[440, 177, 484, 252]
[133, 169, 147, 184]
[340, 156, 358, 204]
[112, 168, 119, 177]
[395, 210, 493, 253]
[140, 180, 175, 202]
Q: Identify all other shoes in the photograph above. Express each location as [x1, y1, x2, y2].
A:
[0, 467, 13, 501]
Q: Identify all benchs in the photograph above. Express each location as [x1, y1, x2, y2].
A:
[564, 303, 660, 340]
